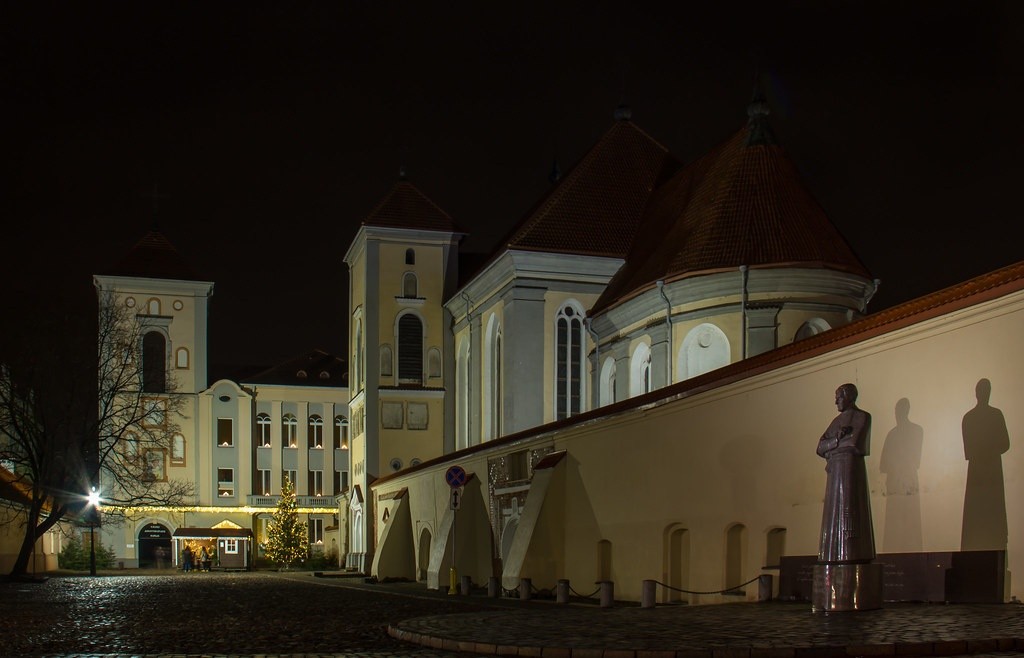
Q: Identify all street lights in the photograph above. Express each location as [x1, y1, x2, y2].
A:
[87, 491, 100, 575]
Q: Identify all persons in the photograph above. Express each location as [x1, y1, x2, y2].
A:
[200, 546, 209, 572]
[154, 546, 165, 569]
[816, 383, 878, 564]
[182, 546, 193, 573]
